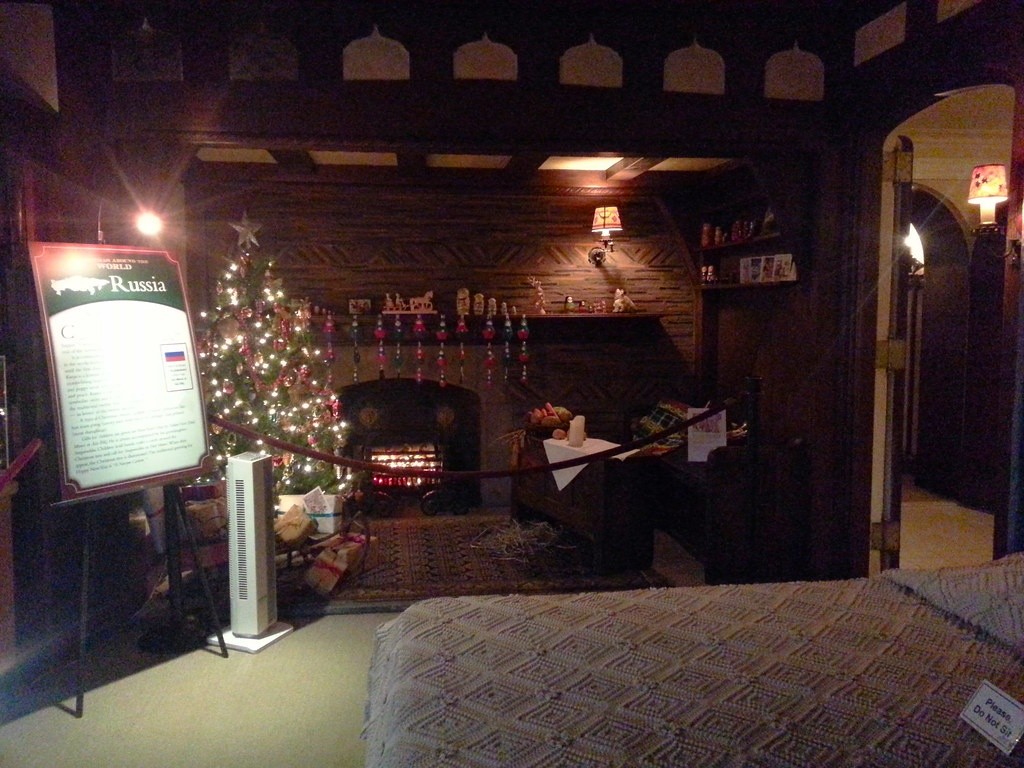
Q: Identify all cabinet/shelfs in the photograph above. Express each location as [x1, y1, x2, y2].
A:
[692, 228, 798, 294]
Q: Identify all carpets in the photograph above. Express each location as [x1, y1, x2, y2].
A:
[131, 510, 717, 620]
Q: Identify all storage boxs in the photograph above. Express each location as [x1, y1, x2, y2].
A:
[273, 493, 378, 597]
[182, 480, 227, 506]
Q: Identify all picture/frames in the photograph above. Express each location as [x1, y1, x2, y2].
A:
[349, 298, 372, 315]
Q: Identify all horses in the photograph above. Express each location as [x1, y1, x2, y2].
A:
[409, 289, 434, 310]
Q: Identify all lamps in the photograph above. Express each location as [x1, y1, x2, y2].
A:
[588, 206, 623, 269]
[968, 162, 1023, 271]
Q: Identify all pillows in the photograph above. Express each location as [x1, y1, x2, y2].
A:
[130, 553, 234, 640]
[184, 496, 233, 545]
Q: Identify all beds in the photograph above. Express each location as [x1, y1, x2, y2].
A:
[358, 547, 1024, 768]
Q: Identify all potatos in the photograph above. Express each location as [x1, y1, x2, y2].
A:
[527, 403, 587, 440]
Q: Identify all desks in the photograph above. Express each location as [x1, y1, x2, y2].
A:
[512, 433, 659, 573]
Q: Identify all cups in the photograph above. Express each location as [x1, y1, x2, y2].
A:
[569, 421, 585, 447]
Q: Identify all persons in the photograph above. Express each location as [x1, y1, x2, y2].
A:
[743, 258, 789, 281]
[386, 293, 408, 308]
[533, 282, 545, 313]
